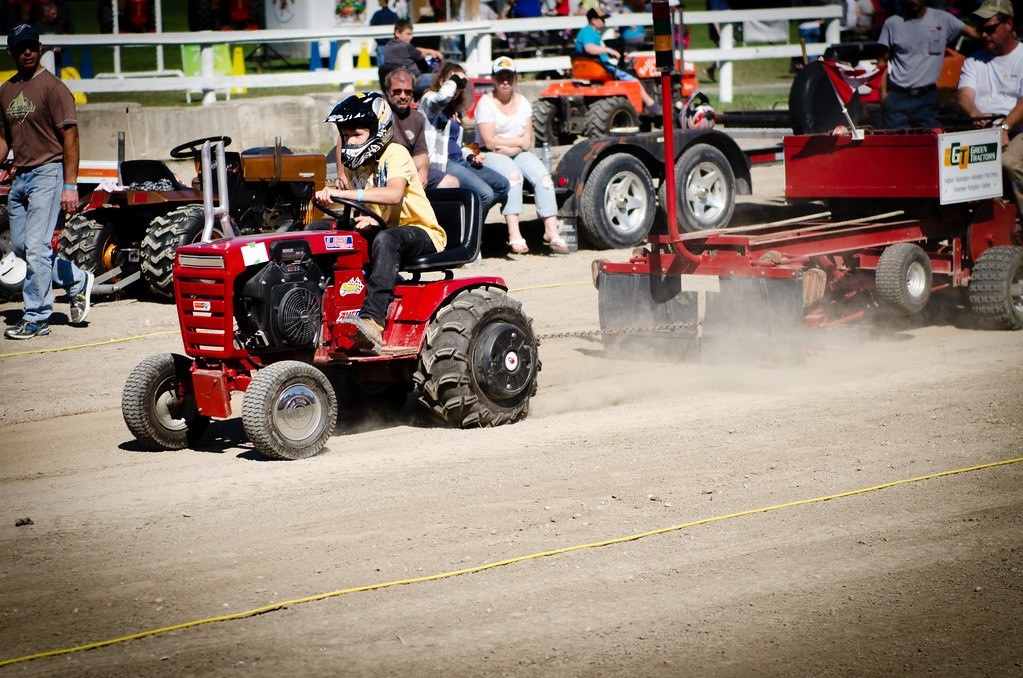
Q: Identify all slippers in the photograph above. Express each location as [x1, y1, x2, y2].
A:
[543, 238, 567, 249]
[509, 238, 530, 253]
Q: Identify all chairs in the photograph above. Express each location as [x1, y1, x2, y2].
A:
[121, 160, 188, 194]
[369, 187, 483, 271]
[570, 52, 619, 85]
[791, 64, 868, 134]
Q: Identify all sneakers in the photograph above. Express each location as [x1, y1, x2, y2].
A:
[4, 319, 51, 339]
[69, 271, 95, 325]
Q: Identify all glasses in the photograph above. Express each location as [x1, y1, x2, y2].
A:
[975, 15, 1008, 35]
[392, 88, 414, 95]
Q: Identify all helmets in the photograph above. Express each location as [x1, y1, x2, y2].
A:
[323, 92, 396, 171]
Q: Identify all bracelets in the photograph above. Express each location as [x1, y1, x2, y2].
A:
[62, 185, 79, 191]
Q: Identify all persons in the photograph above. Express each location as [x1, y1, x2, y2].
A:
[369, 1, 691, 118]
[31, 6, 67, 81]
[474, 56, 568, 253]
[794, 0, 875, 74]
[704, 0, 734, 82]
[1, 25, 94, 341]
[872, 0, 983, 130]
[416, 64, 508, 224]
[304, 92, 447, 355]
[381, 68, 429, 195]
[956, 0, 1023, 243]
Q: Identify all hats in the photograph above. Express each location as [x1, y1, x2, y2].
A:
[586, 6, 611, 19]
[492, 56, 515, 74]
[973, 0, 1014, 19]
[7, 24, 39, 50]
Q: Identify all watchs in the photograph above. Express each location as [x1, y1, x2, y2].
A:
[997, 122, 1010, 131]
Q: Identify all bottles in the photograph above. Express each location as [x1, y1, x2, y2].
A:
[541, 142, 553, 172]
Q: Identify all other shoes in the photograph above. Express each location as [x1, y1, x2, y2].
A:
[339, 313, 384, 356]
[645, 101, 663, 116]
